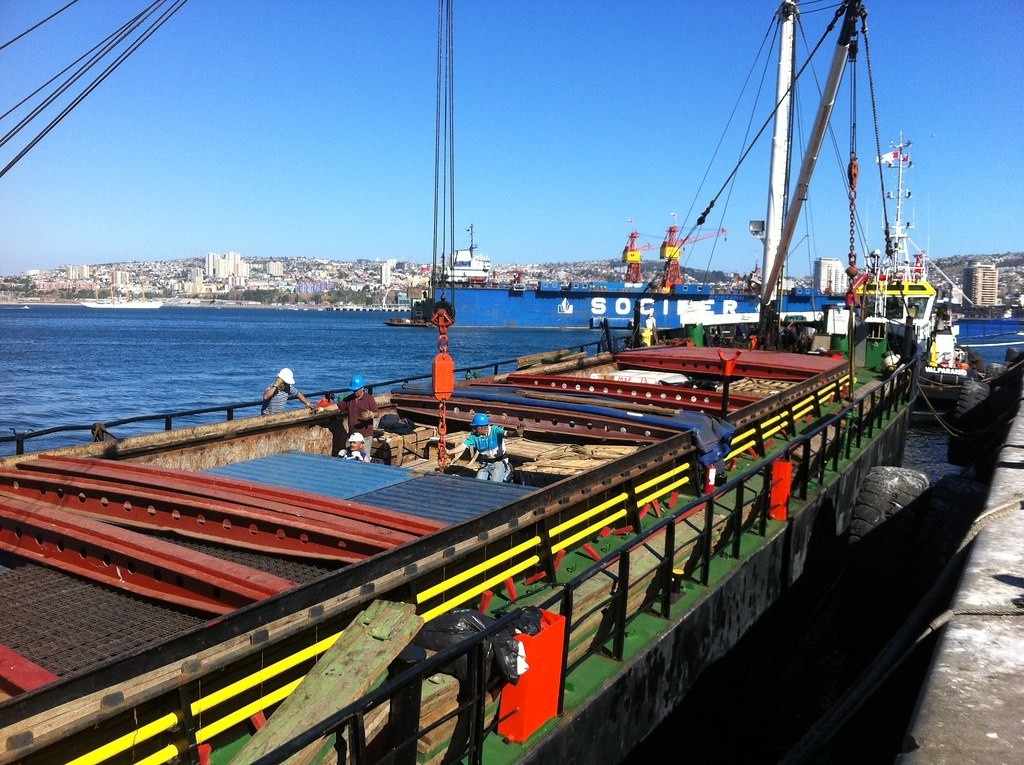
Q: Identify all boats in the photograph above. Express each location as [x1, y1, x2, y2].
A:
[0, 322, 922, 765]
[81, 297, 166, 309]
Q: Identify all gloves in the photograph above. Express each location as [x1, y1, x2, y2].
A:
[351, 450, 363, 461]
[273, 377, 283, 387]
[310, 406, 324, 414]
[308, 402, 317, 412]
[338, 450, 347, 457]
[359, 409, 374, 421]
[515, 423, 526, 438]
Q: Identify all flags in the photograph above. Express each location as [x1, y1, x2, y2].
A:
[671, 212, 676, 217]
[628, 219, 632, 222]
[876, 151, 908, 164]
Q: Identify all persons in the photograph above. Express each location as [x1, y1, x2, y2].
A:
[338, 433, 370, 462]
[956, 360, 969, 369]
[317, 375, 379, 457]
[445, 413, 526, 482]
[261, 367, 317, 416]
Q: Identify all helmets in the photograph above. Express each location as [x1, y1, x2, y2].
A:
[348, 374, 365, 391]
[278, 368, 296, 385]
[470, 413, 490, 426]
[349, 432, 364, 442]
